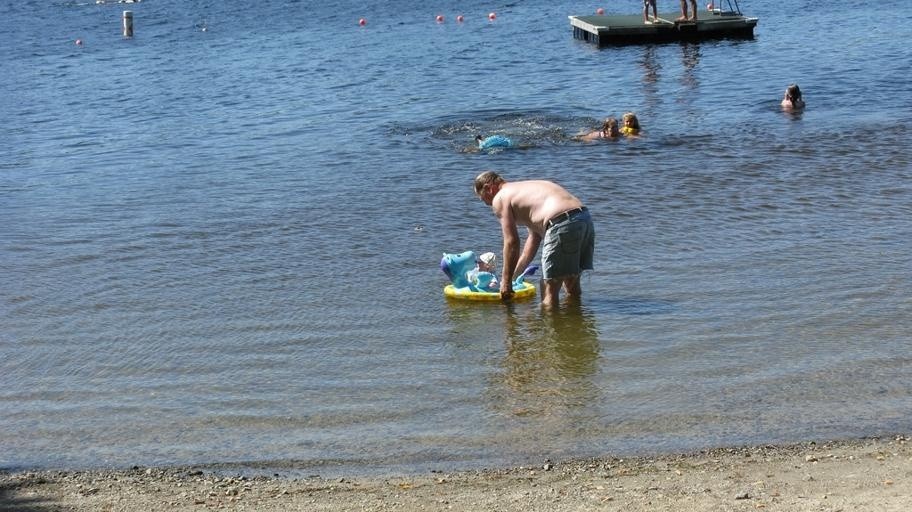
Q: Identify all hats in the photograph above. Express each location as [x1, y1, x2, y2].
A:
[477, 252, 498, 269]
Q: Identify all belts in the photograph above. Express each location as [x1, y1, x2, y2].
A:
[546, 206, 587, 230]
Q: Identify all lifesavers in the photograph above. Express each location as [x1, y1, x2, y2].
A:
[479, 135, 511, 148]
[443, 280, 536, 302]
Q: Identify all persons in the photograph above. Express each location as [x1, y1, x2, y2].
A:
[618, 114, 645, 139]
[475, 170, 594, 308]
[467, 252, 498, 289]
[782, 84, 805, 110]
[643, 0, 661, 24]
[676, 0, 697, 21]
[575, 118, 618, 142]
